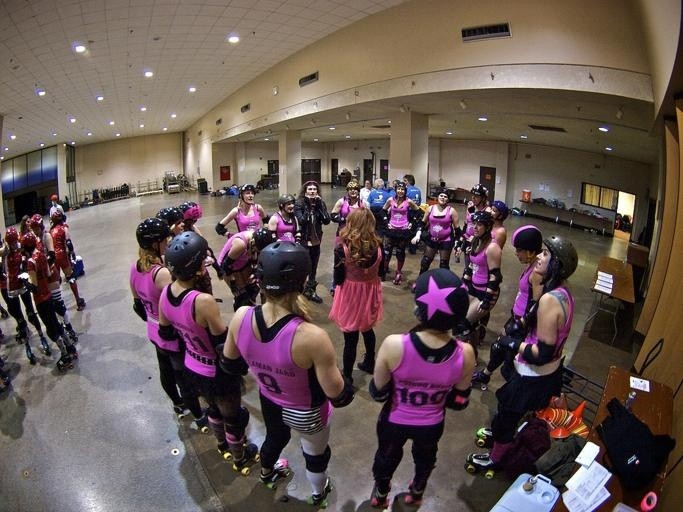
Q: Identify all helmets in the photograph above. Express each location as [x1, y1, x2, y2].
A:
[471, 209, 492, 228]
[164, 231, 209, 282]
[395, 182, 408, 192]
[239, 184, 256, 198]
[540, 234, 579, 281]
[470, 184, 489, 204]
[416, 268, 470, 327]
[136, 201, 202, 249]
[346, 181, 360, 191]
[277, 193, 295, 208]
[255, 240, 313, 297]
[437, 188, 450, 200]
[491, 201, 508, 224]
[512, 224, 544, 256]
[5, 194, 66, 253]
[253, 226, 272, 249]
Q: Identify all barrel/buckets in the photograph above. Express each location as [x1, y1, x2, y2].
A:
[490, 473, 560, 511]
[521, 189, 532, 202]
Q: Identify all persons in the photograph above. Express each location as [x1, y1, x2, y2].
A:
[130, 201, 215, 433]
[223, 242, 355, 510]
[158, 231, 261, 477]
[465, 225, 578, 480]
[328, 206, 382, 383]
[0, 194, 86, 393]
[331, 175, 462, 291]
[358, 268, 476, 511]
[219, 182, 331, 311]
[454, 184, 509, 366]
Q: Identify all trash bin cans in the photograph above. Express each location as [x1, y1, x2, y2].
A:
[197, 178, 208, 195]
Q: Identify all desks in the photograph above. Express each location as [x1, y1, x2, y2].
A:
[623, 260, 644, 303]
[259, 174, 279, 189]
[517, 199, 567, 223]
[582, 255, 637, 347]
[568, 209, 613, 236]
[541, 364, 674, 511]
[334, 174, 358, 187]
[444, 187, 472, 203]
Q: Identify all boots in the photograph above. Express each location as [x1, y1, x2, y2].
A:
[358, 353, 375, 374]
[304, 280, 323, 302]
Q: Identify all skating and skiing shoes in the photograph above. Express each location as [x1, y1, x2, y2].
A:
[405, 479, 424, 506]
[470, 368, 490, 391]
[475, 426, 494, 447]
[393, 270, 403, 284]
[258, 458, 290, 490]
[411, 283, 416, 292]
[307, 476, 333, 508]
[370, 480, 392, 509]
[0, 298, 86, 390]
[194, 408, 209, 433]
[464, 451, 496, 480]
[233, 443, 260, 476]
[174, 398, 188, 420]
[216, 434, 248, 461]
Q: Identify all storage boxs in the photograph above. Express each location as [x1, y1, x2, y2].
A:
[626, 241, 649, 269]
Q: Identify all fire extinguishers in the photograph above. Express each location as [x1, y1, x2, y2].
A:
[614, 213, 623, 230]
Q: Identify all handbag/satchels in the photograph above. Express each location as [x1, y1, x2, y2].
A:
[210, 183, 238, 198]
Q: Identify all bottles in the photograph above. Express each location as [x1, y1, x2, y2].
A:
[623, 391, 636, 409]
[639, 491, 657, 511]
